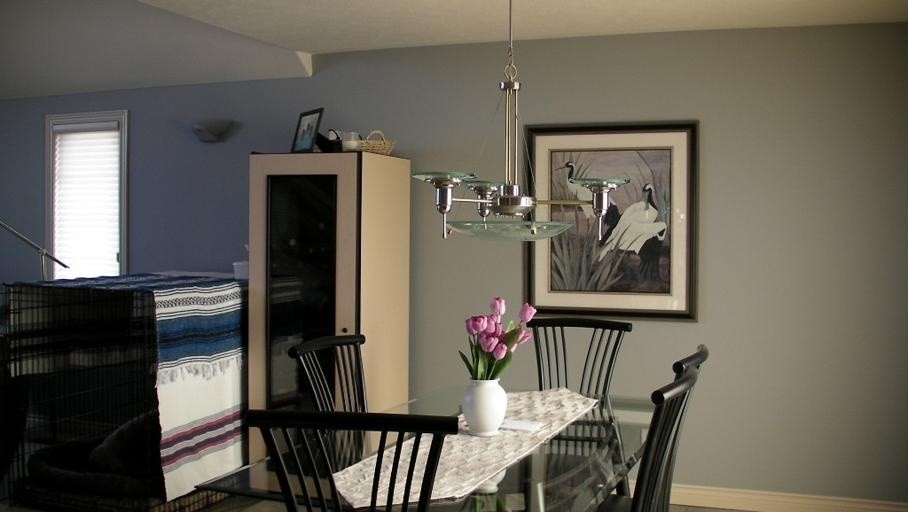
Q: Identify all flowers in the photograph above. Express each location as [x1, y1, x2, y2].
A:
[458, 296, 537, 380]
[459, 489, 512, 512]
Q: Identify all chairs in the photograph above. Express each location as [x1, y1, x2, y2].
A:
[245, 410, 458, 512]
[496, 318, 632, 512]
[287, 335, 368, 413]
[595, 345, 709, 512]
[630, 366, 698, 512]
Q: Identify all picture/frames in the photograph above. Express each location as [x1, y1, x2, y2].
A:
[290, 108, 324, 153]
[523, 119, 699, 321]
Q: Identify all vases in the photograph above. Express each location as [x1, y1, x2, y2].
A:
[475, 468, 507, 494]
[461, 378, 508, 437]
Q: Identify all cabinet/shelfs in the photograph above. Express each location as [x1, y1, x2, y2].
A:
[248, 151, 410, 503]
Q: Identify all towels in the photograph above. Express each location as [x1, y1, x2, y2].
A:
[332, 387, 599, 508]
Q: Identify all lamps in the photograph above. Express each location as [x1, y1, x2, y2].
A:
[410, 1, 626, 242]
